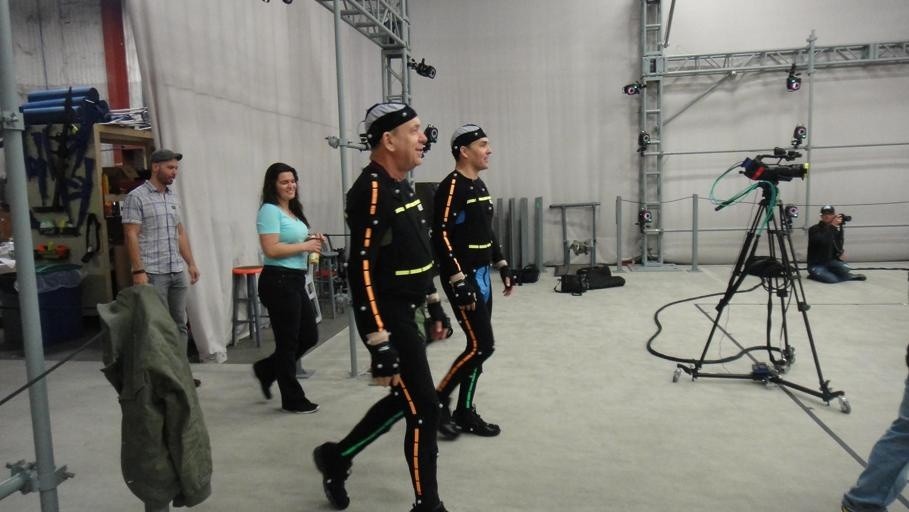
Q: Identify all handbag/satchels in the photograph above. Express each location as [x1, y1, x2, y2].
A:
[561, 273, 583, 292]
[523, 263, 539, 283]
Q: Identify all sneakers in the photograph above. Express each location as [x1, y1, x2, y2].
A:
[312, 442, 350, 507]
[281, 400, 318, 414]
[454, 406, 502, 436]
[435, 388, 462, 438]
[252, 360, 273, 398]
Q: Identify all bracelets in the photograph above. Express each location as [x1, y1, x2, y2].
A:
[131, 269, 146, 274]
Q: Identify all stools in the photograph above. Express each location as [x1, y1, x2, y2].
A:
[231, 265, 266, 346]
[313, 251, 345, 318]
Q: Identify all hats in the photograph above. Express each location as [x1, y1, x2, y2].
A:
[849, 273, 865, 280]
[149, 147, 183, 162]
[365, 103, 418, 142]
[451, 122, 485, 150]
[820, 204, 835, 215]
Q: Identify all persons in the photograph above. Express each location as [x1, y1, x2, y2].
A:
[840, 344, 909, 512]
[430, 125, 516, 440]
[252, 163, 325, 415]
[311, 102, 453, 512]
[122, 149, 201, 387]
[807, 205, 866, 283]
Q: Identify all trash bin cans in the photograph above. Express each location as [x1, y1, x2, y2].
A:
[15, 260, 88, 347]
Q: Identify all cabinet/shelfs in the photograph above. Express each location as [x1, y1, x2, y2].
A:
[94, 126, 155, 311]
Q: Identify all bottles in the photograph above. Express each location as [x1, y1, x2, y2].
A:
[310, 235, 322, 267]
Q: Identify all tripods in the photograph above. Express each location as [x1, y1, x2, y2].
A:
[671, 205, 852, 415]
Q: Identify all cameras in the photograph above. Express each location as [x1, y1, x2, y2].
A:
[837, 212, 852, 225]
